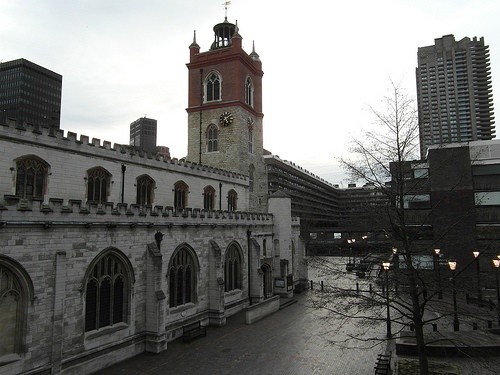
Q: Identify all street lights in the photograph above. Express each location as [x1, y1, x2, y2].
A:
[392, 245, 400, 293]
[472, 250, 482, 307]
[448, 260, 461, 332]
[382, 262, 393, 339]
[491, 259, 500, 325]
[434, 247, 443, 300]
[346, 234, 367, 268]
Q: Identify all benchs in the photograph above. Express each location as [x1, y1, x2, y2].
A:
[182, 320, 206, 343]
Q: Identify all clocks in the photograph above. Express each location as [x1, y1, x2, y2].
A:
[219, 112, 234, 126]
